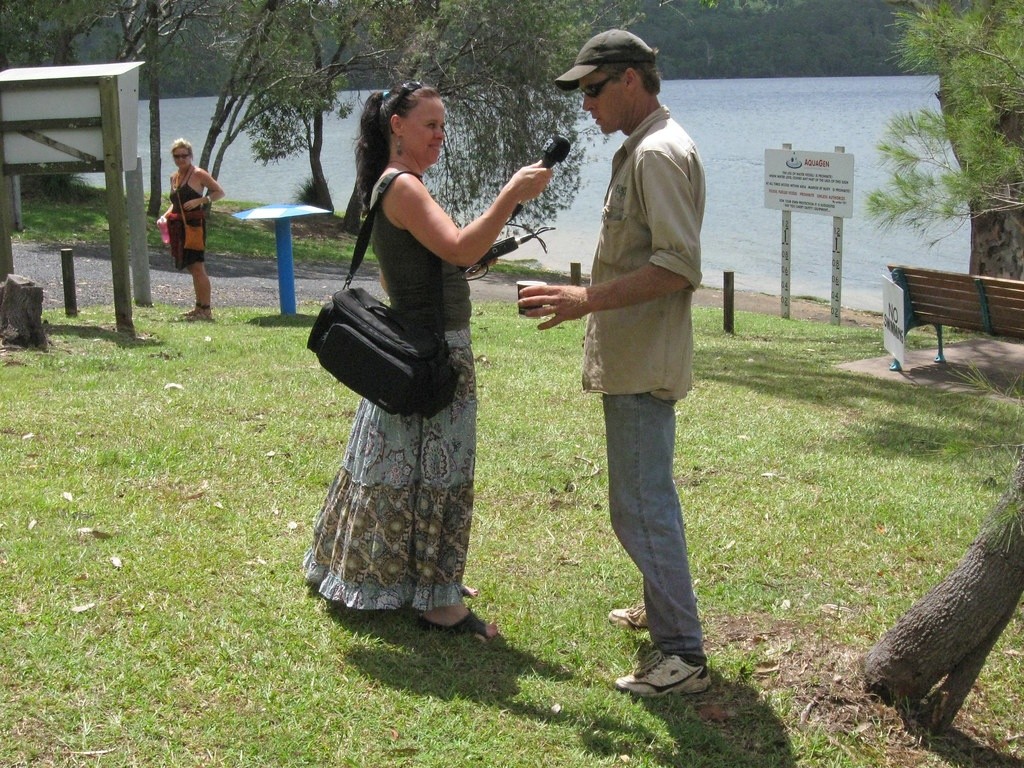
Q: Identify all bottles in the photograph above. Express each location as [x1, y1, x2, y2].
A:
[157, 219, 170, 244]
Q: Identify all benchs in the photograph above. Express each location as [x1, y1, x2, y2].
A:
[887, 263, 1024, 372]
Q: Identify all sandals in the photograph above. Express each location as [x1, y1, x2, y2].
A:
[187, 306, 213, 322]
[182, 303, 202, 316]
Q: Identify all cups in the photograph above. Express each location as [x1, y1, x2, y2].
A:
[516, 280, 547, 319]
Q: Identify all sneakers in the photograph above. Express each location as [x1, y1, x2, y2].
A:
[613, 653, 710, 697]
[608, 604, 648, 630]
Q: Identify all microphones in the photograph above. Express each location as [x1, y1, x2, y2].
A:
[511, 135, 571, 219]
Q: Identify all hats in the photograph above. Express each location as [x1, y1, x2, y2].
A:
[554, 29, 655, 92]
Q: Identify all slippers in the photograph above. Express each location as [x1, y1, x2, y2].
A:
[417, 606, 499, 642]
[454, 583, 479, 599]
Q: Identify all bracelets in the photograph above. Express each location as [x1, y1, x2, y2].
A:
[206, 195, 211, 204]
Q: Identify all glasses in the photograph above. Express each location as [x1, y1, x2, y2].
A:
[387, 81, 422, 135]
[581, 75, 616, 98]
[172, 154, 190, 159]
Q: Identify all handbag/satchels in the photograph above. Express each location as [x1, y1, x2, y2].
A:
[183, 225, 205, 251]
[307, 290, 463, 419]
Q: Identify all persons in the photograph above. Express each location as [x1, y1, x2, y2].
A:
[302, 82, 552, 640]
[157, 138, 224, 321]
[516, 28, 709, 700]
